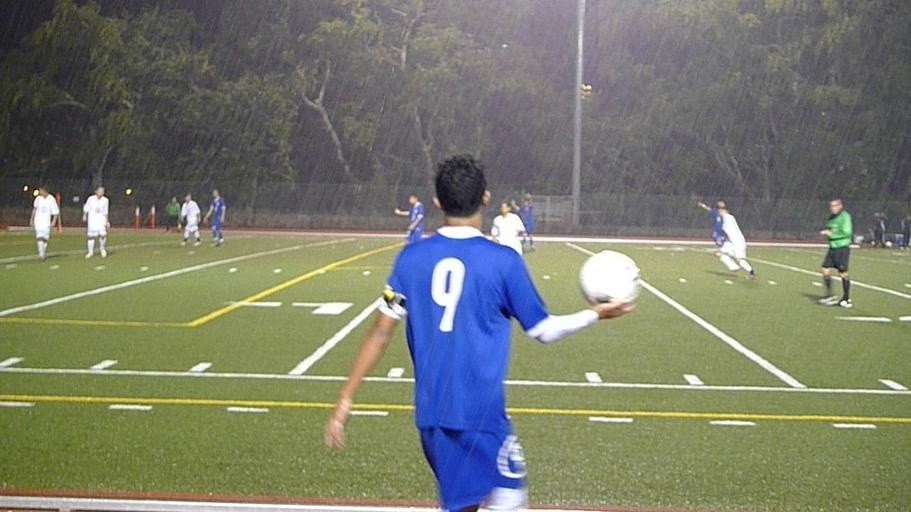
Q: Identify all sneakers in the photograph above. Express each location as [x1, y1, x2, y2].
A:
[86, 249, 107, 259]
[819, 295, 851, 309]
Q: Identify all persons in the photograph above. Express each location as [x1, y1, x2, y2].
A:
[202, 187, 226, 244]
[29, 183, 60, 261]
[179, 191, 202, 246]
[900, 212, 911, 251]
[719, 206, 754, 274]
[82, 185, 111, 258]
[326, 151, 640, 510]
[816, 196, 853, 309]
[511, 191, 536, 252]
[698, 200, 729, 248]
[870, 211, 889, 248]
[395, 194, 425, 245]
[490, 200, 529, 262]
[51, 191, 61, 227]
[164, 194, 181, 233]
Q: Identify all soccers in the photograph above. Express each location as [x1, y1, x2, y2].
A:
[578, 249, 641, 305]
